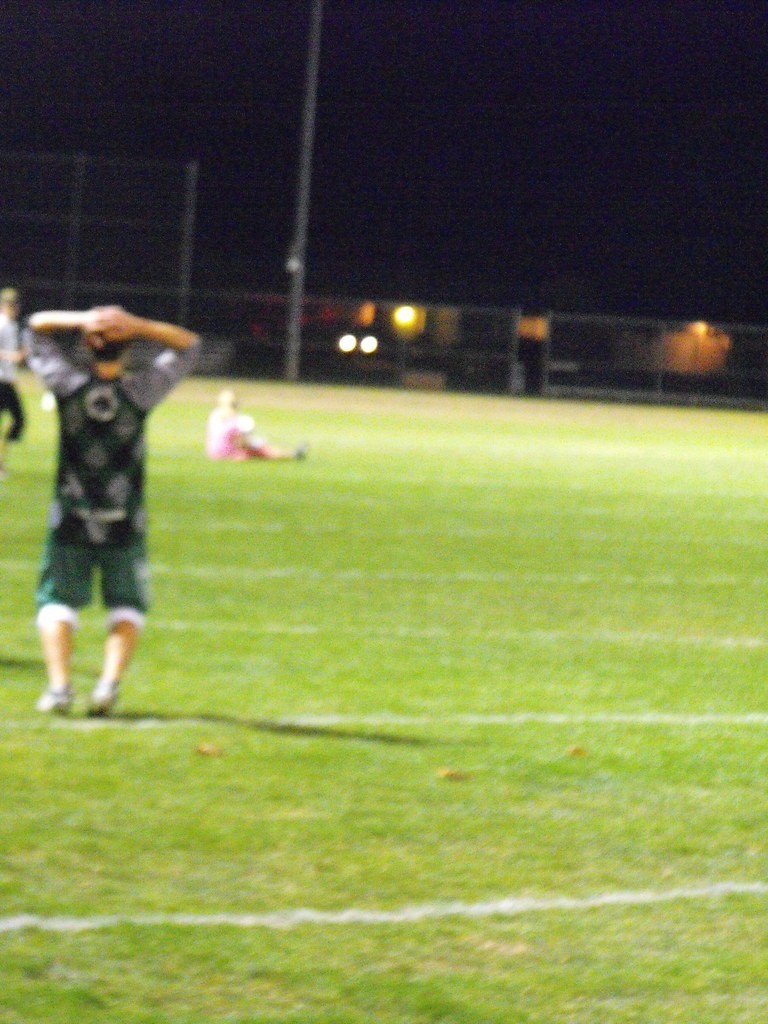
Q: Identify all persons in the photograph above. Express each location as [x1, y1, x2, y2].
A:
[20, 305, 200, 716]
[0, 286, 310, 463]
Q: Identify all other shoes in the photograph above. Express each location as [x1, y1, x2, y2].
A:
[37, 691, 74, 716]
[89, 681, 118, 716]
[294, 447, 309, 462]
